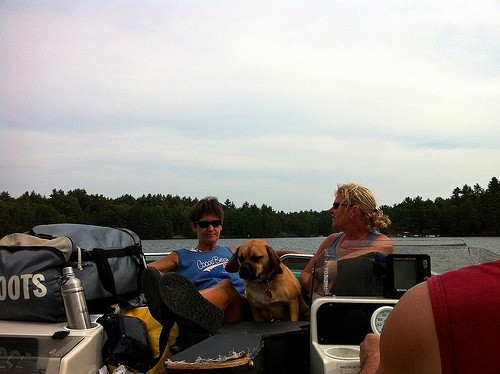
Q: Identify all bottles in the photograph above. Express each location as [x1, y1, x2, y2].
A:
[59, 267, 92, 330]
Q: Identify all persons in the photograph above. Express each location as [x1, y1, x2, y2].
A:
[358, 259, 500, 374]
[140, 194, 311, 334]
[299, 183, 394, 302]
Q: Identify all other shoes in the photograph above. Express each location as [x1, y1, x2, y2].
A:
[141, 266, 164, 305]
[158, 272, 223, 332]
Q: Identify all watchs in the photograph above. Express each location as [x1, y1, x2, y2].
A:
[311, 267, 320, 276]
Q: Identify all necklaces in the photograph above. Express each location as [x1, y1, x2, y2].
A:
[194, 248, 202, 252]
[341, 232, 367, 252]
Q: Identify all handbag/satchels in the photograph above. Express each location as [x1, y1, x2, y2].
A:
[0, 224, 149, 323]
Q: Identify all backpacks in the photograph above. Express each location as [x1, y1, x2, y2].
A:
[96, 305, 178, 374]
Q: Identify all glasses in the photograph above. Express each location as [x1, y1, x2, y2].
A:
[333, 202, 352, 209]
[195, 220, 222, 228]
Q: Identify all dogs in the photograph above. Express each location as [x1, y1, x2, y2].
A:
[225, 238, 311, 321]
